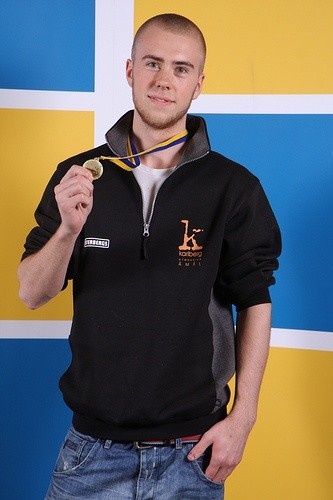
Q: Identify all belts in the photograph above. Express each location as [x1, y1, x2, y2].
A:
[136, 434, 202, 444]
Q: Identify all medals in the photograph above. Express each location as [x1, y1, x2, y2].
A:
[83, 158, 103, 181]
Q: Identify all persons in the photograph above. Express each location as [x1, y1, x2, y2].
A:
[17, 13, 282, 500]
